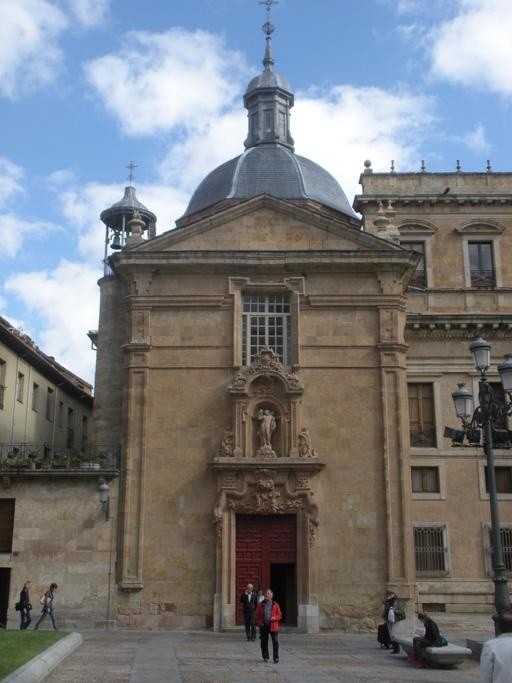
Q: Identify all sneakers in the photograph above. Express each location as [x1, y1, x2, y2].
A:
[264, 658, 269, 662]
[390, 649, 400, 654]
[247, 637, 255, 641]
[407, 655, 417, 661]
[416, 663, 426, 668]
[274, 657, 279, 663]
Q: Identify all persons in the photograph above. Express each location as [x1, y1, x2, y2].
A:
[479, 609, 512, 683]
[18, 580, 32, 630]
[35, 581, 59, 630]
[380, 590, 404, 654]
[254, 408, 277, 446]
[241, 583, 283, 663]
[404, 612, 442, 669]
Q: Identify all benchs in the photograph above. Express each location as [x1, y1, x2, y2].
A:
[395, 634, 472, 669]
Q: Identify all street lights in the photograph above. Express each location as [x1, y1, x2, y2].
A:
[445, 334, 511, 637]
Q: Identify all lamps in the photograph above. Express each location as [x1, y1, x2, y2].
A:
[97, 474, 112, 521]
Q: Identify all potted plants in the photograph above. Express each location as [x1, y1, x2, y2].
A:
[0, 441, 103, 471]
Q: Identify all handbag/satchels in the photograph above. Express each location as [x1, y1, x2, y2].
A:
[40, 595, 51, 606]
[394, 609, 406, 622]
[440, 634, 448, 646]
[16, 602, 32, 611]
[377, 624, 392, 644]
